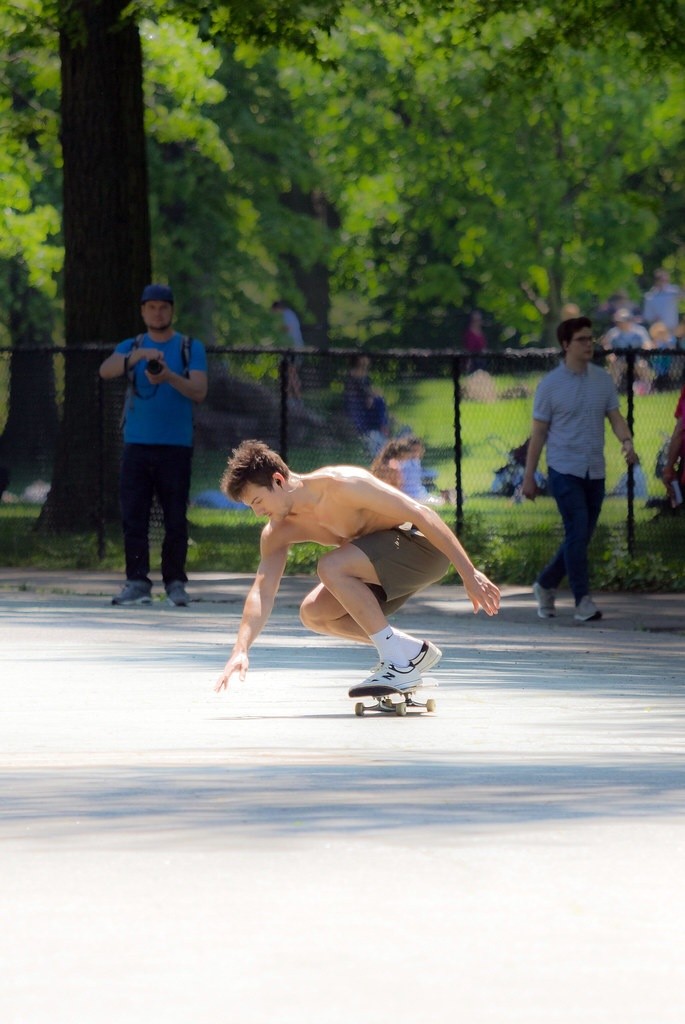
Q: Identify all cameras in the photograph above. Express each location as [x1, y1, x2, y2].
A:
[147, 358, 163, 375]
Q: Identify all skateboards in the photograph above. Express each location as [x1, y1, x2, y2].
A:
[349, 678, 439, 716]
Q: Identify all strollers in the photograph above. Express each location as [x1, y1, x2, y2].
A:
[485, 432, 549, 496]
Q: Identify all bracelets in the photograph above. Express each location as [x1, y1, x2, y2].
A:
[623, 438, 633, 444]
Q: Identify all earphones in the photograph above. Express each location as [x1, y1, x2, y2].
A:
[277, 479, 281, 486]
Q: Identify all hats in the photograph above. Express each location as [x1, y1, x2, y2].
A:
[141, 285, 174, 302]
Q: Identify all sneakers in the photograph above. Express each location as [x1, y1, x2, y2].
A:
[408, 639, 442, 673]
[112, 585, 153, 606]
[167, 581, 190, 607]
[533, 583, 556, 617]
[348, 661, 422, 697]
[573, 596, 602, 621]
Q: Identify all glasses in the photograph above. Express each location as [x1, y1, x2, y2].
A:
[570, 335, 593, 344]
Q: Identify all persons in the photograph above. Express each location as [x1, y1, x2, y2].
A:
[492, 441, 550, 501]
[521, 317, 639, 621]
[271, 302, 305, 389]
[552, 268, 685, 394]
[663, 383, 685, 513]
[213, 439, 501, 688]
[344, 355, 445, 505]
[610, 451, 648, 496]
[464, 308, 487, 368]
[97, 283, 207, 607]
